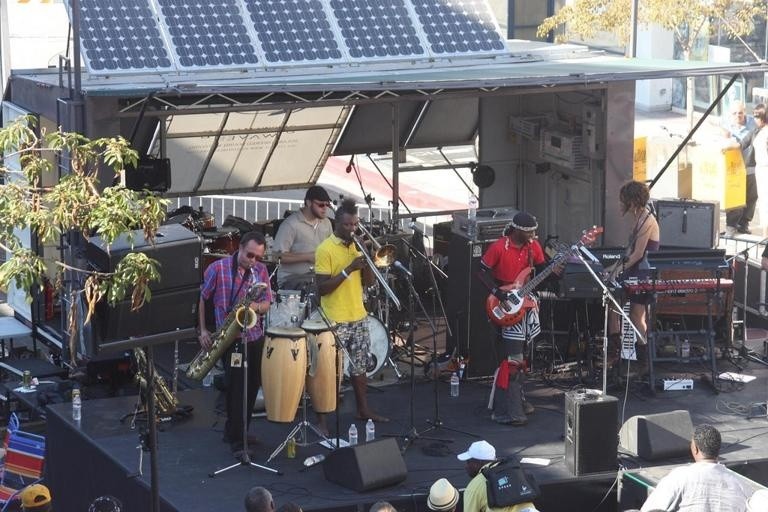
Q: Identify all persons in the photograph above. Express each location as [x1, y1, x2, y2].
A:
[640, 423, 752, 512]
[16, 482, 54, 512]
[477, 210, 567, 417]
[607, 178, 661, 379]
[273, 185, 333, 309]
[370, 500, 397, 512]
[244, 486, 273, 511]
[314, 196, 391, 438]
[458, 440, 542, 512]
[279, 503, 304, 511]
[716, 95, 768, 275]
[195, 230, 273, 461]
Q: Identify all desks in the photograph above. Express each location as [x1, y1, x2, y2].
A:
[0, 376, 94, 422]
[0, 359, 67, 379]
[0, 317, 33, 359]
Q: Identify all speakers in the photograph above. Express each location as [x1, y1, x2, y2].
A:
[324, 437, 409, 494]
[619, 409, 693, 461]
[563, 391, 619, 477]
[81, 220, 203, 340]
[125, 158, 171, 191]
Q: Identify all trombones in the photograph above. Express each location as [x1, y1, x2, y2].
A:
[350, 220, 404, 311]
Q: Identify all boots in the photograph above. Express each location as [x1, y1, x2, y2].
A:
[635, 341, 649, 380]
[607, 333, 621, 368]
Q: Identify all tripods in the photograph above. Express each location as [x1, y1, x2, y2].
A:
[416, 290, 484, 439]
[209, 285, 286, 477]
[379, 279, 455, 454]
[264, 381, 340, 464]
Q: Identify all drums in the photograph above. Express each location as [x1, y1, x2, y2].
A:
[204, 225, 240, 256]
[268, 290, 312, 329]
[166, 214, 201, 236]
[260, 325, 306, 422]
[302, 318, 343, 414]
[339, 312, 392, 380]
[192, 211, 216, 231]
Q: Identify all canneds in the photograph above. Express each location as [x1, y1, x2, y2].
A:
[23, 370, 31, 388]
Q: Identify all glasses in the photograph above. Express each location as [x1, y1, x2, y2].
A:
[312, 200, 330, 207]
[247, 253, 263, 261]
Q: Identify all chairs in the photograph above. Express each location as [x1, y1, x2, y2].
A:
[0, 413, 19, 463]
[0, 430, 47, 512]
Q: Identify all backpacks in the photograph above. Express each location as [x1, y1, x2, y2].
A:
[482, 456, 541, 508]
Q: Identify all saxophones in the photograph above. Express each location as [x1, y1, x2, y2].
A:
[185, 261, 268, 382]
[124, 338, 180, 420]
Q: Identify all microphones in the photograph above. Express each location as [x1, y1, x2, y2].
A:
[570, 242, 585, 263]
[298, 286, 307, 310]
[345, 153, 355, 173]
[393, 260, 416, 278]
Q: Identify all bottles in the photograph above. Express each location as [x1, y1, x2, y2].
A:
[366, 420, 375, 441]
[287, 437, 296, 458]
[349, 424, 359, 448]
[451, 372, 460, 396]
[73, 394, 81, 421]
[303, 453, 325, 466]
[682, 339, 690, 356]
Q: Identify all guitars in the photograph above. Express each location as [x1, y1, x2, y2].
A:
[485, 223, 603, 327]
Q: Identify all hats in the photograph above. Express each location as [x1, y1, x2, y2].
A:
[22, 484, 52, 507]
[457, 440, 496, 461]
[306, 186, 330, 201]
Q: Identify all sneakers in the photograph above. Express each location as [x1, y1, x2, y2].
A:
[224, 434, 255, 458]
[512, 401, 535, 426]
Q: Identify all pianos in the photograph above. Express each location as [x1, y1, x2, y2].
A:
[545, 242, 623, 298]
[621, 277, 734, 294]
[619, 299, 639, 361]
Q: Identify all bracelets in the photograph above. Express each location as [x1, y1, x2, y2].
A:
[490, 286, 498, 295]
[255, 303, 261, 312]
[342, 268, 350, 279]
